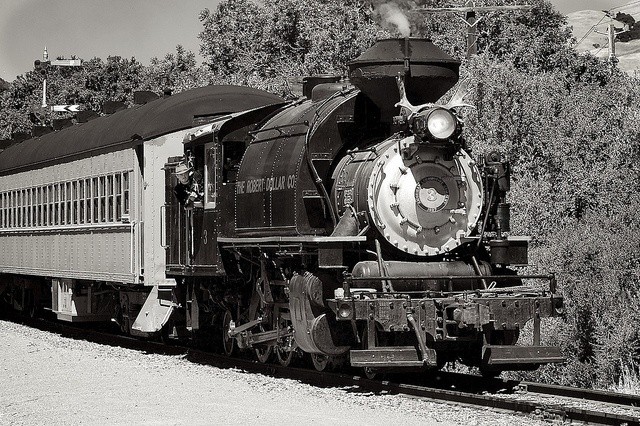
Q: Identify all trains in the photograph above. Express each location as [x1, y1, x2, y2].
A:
[0, 35, 567, 381]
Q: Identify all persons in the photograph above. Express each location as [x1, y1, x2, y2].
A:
[173, 164, 202, 207]
